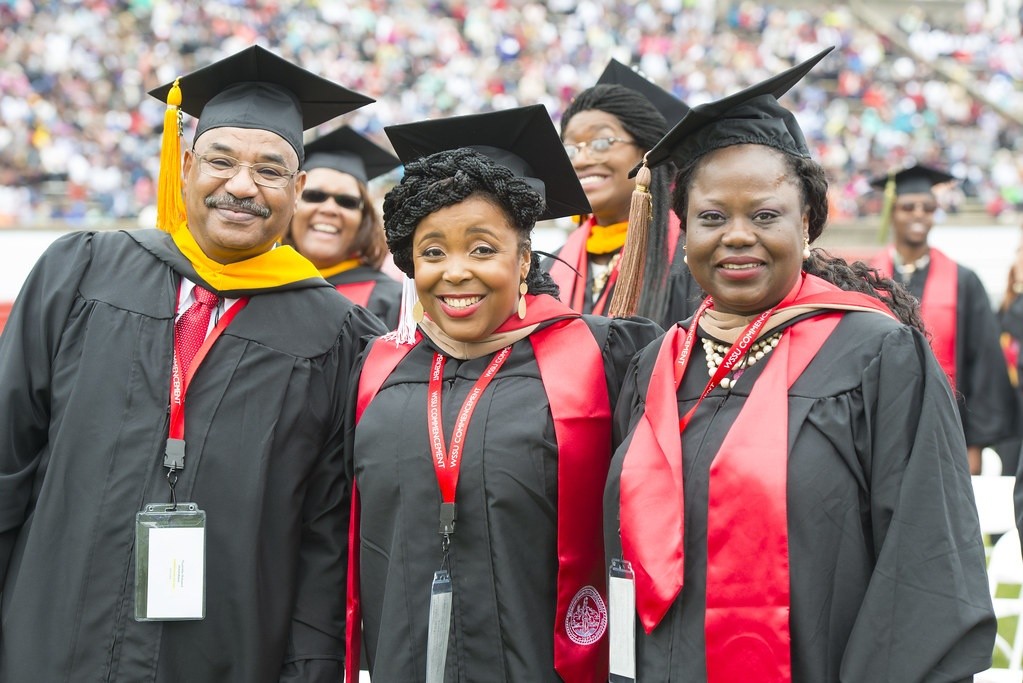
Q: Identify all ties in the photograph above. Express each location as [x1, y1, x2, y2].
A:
[176, 284, 219, 383]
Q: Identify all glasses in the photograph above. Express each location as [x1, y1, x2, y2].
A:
[301, 189, 364, 209]
[192, 149, 299, 188]
[564, 138, 637, 160]
[894, 200, 937, 213]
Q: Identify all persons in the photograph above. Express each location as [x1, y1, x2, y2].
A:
[282, 124, 403, 330]
[1, 45, 376, 683]
[602, 44, 999, 683]
[995, 246, 1023, 558]
[539, 61, 705, 331]
[849, 163, 1017, 475]
[0, 1, 1023, 232]
[344, 103, 664, 683]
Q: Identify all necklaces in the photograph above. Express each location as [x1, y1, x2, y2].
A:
[701, 332, 783, 389]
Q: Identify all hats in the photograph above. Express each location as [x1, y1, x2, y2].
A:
[147, 44, 377, 240]
[302, 124, 403, 185]
[869, 164, 955, 245]
[597, 57, 691, 130]
[384, 104, 594, 348]
[609, 45, 835, 319]
